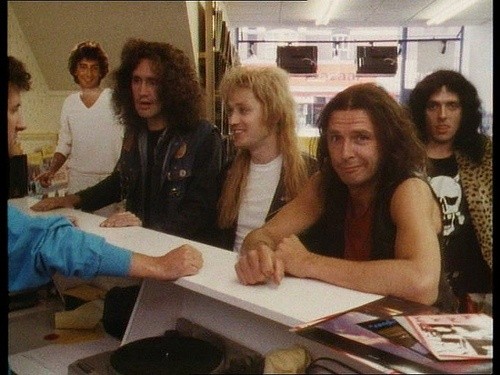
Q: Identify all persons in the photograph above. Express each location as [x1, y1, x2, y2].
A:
[203, 65, 319, 254]
[35, 41, 126, 216]
[8, 55, 203, 294]
[30, 37, 223, 246]
[234, 82, 456, 314]
[409, 70, 493, 295]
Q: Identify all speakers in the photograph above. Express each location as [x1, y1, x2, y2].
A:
[9, 154, 28, 199]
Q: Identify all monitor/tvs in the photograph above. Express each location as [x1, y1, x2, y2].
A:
[276, 45, 318, 77]
[354, 44, 398, 75]
